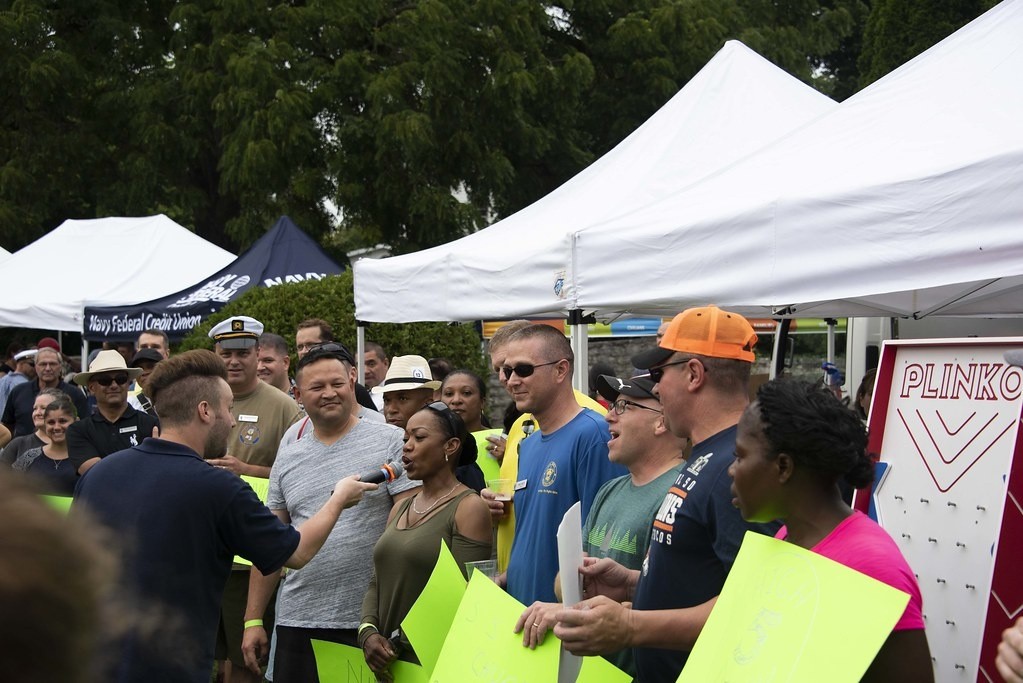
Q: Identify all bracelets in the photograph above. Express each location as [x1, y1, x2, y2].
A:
[243, 619, 263, 629]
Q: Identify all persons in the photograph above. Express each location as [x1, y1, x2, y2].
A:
[480, 319, 629, 605]
[555, 305, 936, 683]
[1, 317, 491, 682]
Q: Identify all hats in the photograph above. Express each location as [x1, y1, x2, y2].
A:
[73, 349, 144, 386]
[596, 374, 656, 403]
[631, 304, 758, 370]
[37, 338, 60, 353]
[207, 316, 265, 349]
[371, 355, 443, 394]
[87, 348, 103, 365]
[128, 349, 164, 366]
[13, 350, 38, 362]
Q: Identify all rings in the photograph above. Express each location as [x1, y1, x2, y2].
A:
[533, 623, 539, 628]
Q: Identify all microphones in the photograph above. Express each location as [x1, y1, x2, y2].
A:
[359, 462, 403, 484]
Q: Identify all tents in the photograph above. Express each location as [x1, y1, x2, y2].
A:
[0, 214, 352, 376]
[355, 0, 1023, 385]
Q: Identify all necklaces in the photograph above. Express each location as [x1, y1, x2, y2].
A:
[412, 481, 463, 514]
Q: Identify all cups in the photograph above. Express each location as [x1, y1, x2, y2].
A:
[464, 560, 496, 583]
[488, 479, 511, 518]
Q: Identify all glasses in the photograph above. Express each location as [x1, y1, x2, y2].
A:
[90, 373, 132, 386]
[24, 361, 35, 367]
[421, 400, 457, 437]
[309, 342, 350, 356]
[648, 360, 709, 384]
[516, 419, 535, 455]
[607, 399, 663, 416]
[654, 331, 663, 338]
[296, 340, 329, 351]
[502, 358, 570, 380]
